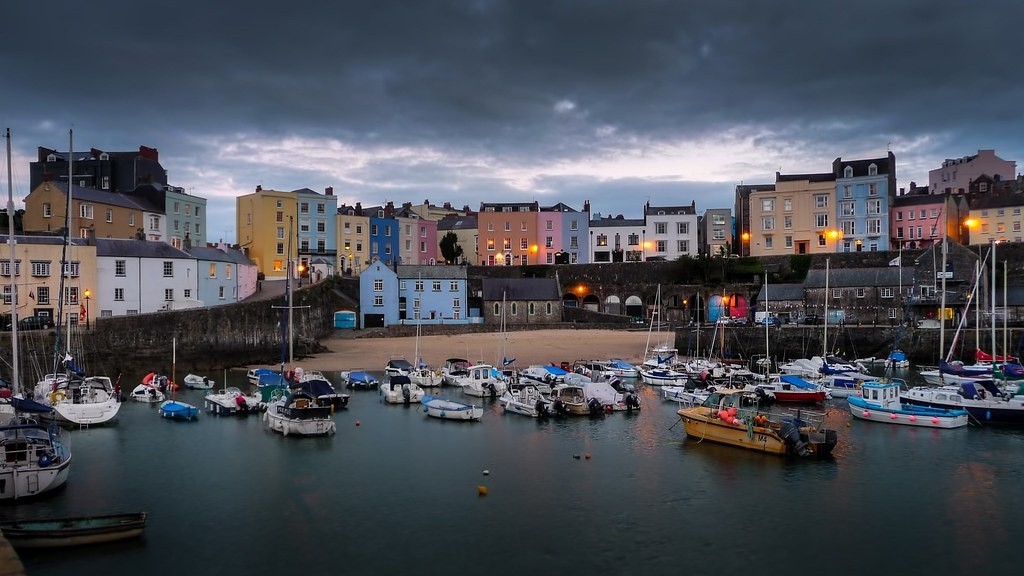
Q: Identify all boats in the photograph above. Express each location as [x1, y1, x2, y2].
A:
[157, 337, 200, 420]
[341, 368, 379, 391]
[183, 372, 215, 390]
[420, 395, 484, 421]
[205, 215, 350, 436]
[643, 199, 1024, 420]
[0, 511, 149, 550]
[386, 272, 643, 430]
[379, 374, 425, 403]
[129, 372, 180, 403]
[846, 379, 969, 428]
[669, 392, 838, 458]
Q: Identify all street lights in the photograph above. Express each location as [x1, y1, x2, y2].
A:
[83, 289, 91, 330]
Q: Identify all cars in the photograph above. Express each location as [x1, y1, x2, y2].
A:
[6, 316, 55, 330]
[762, 317, 781, 329]
[715, 315, 754, 327]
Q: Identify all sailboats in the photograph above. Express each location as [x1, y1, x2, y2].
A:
[0, 128, 72, 500]
[19, 128, 125, 428]
